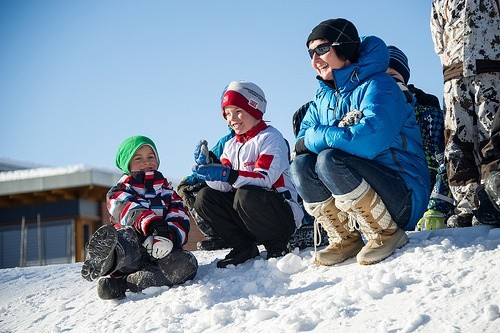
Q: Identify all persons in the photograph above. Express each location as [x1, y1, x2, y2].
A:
[192, 81, 305, 269]
[176, 85, 236, 249]
[382, 44, 456, 231]
[290, 18, 431, 265]
[80, 136, 198, 300]
[430, 0, 500, 228]
[289, 101, 330, 251]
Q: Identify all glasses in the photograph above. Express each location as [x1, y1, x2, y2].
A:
[308, 41, 358, 59]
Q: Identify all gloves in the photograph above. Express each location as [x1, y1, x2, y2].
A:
[142, 219, 180, 259]
[194, 140, 213, 165]
[415, 210, 447, 232]
[192, 163, 239, 186]
[337, 110, 362, 127]
[294, 137, 310, 155]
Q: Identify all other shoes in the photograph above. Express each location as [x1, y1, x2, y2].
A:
[446, 214, 473, 228]
[196, 237, 226, 250]
[217, 245, 259, 268]
[471, 215, 479, 226]
[289, 224, 329, 250]
[266, 245, 288, 259]
[97, 278, 128, 300]
[81, 224, 118, 282]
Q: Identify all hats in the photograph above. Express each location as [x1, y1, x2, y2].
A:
[115, 135, 160, 174]
[387, 46, 410, 84]
[220, 80, 267, 120]
[307, 18, 360, 63]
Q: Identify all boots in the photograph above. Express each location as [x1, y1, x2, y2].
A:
[332, 178, 409, 264]
[302, 196, 365, 265]
